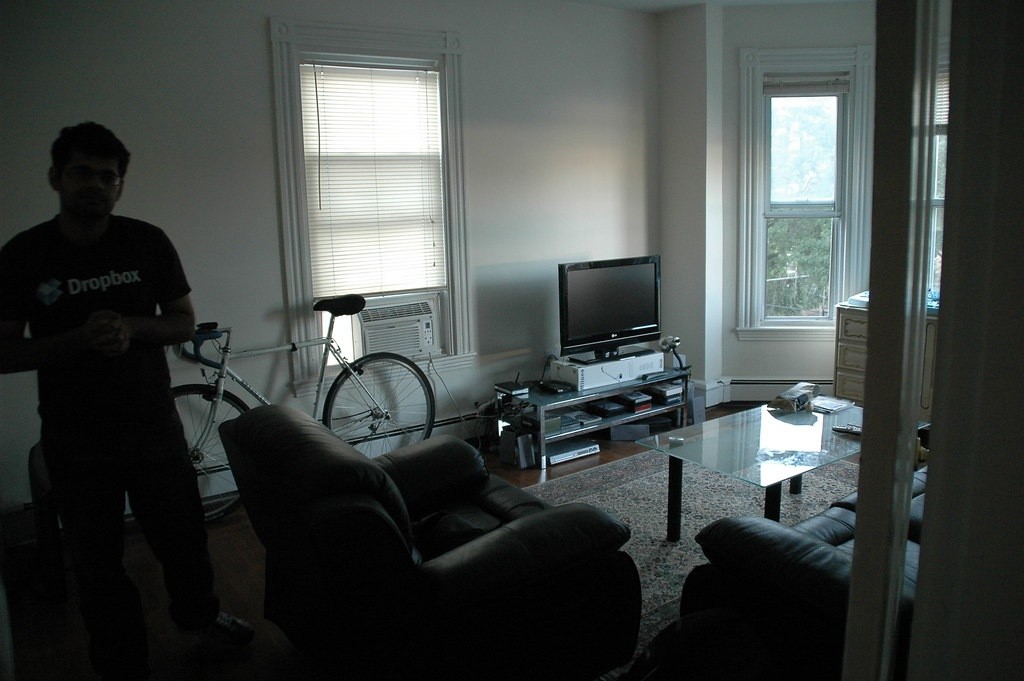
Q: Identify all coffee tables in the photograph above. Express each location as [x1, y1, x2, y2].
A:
[635, 404, 932, 543]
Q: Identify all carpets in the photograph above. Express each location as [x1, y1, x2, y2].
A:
[518, 421, 860, 680]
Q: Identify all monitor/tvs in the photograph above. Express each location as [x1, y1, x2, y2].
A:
[558, 255, 661, 364]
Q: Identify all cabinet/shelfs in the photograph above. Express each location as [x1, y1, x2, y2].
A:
[495, 369, 692, 471]
[834, 293, 937, 422]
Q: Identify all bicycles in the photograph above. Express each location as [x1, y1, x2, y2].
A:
[168, 292, 435, 523]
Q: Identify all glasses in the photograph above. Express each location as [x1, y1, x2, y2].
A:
[61, 171, 123, 186]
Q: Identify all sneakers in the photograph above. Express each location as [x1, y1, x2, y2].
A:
[209, 610, 256, 643]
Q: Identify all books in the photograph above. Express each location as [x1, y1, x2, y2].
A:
[812, 395, 855, 414]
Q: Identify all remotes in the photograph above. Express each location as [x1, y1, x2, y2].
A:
[832, 424, 862, 435]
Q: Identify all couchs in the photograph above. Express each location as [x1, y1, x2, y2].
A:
[681, 464, 927, 681]
[218, 402, 642, 681]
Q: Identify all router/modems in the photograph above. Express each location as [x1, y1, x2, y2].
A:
[495, 372, 529, 395]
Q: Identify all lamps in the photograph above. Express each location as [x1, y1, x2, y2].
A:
[661, 335, 692, 370]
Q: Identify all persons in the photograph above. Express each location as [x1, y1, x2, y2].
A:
[0, 121, 254, 681]
